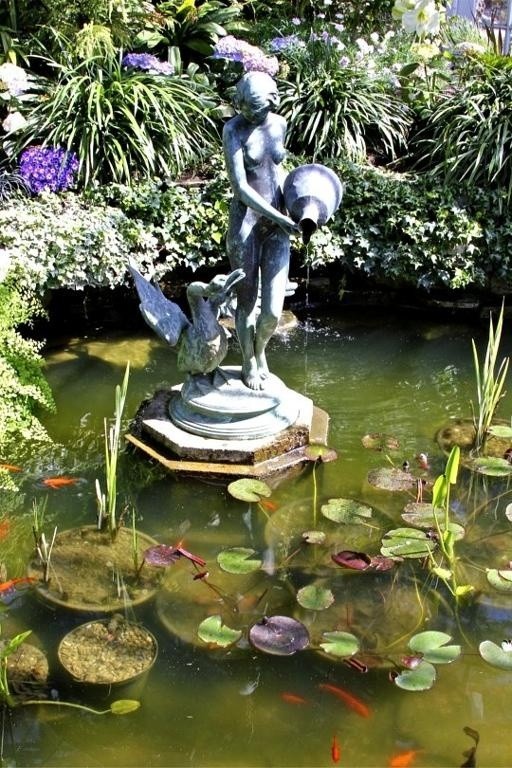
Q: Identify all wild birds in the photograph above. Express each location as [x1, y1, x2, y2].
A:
[126, 265, 248, 396]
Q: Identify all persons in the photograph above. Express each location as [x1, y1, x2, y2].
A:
[222, 71, 299, 390]
